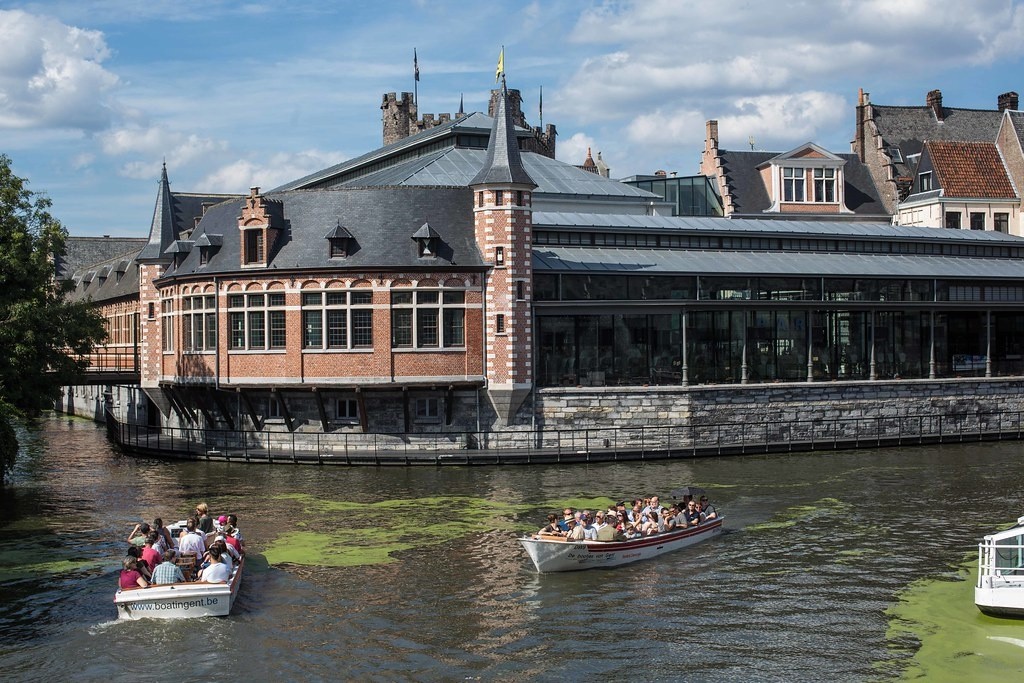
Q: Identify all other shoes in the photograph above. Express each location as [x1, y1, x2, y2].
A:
[191, 566, 199, 578]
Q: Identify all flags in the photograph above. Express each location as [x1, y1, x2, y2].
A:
[496, 49, 503, 82]
[414, 54, 419, 81]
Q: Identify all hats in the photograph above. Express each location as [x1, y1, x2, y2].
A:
[580, 514, 588, 520]
[606, 511, 617, 517]
[564, 515, 575, 524]
[218, 516, 228, 524]
[616, 501, 624, 507]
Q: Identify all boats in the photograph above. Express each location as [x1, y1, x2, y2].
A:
[953, 354, 988, 371]
[517, 510, 726, 571]
[114, 519, 245, 620]
[974, 515, 1024, 620]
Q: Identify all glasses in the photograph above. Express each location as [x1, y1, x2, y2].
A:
[566, 512, 573, 515]
[702, 499, 708, 501]
[696, 507, 701, 508]
[689, 504, 694, 506]
[595, 516, 600, 518]
[620, 504, 624, 506]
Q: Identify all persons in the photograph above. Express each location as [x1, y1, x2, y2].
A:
[538, 495, 717, 542]
[119, 502, 245, 588]
